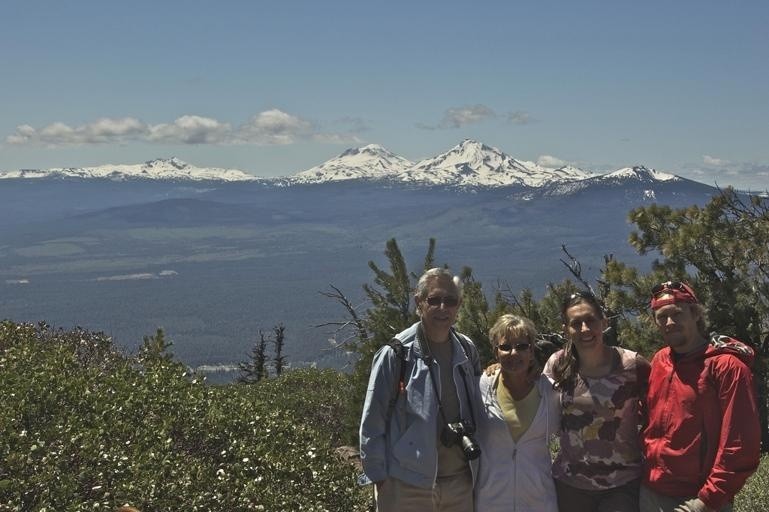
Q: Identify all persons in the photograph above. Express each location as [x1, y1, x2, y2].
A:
[470, 314, 565, 512]
[638, 280, 761, 512]
[355, 269, 481, 512]
[486, 291, 652, 512]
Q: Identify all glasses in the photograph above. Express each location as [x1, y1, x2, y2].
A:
[651, 281, 683, 292]
[425, 296, 459, 306]
[494, 343, 530, 352]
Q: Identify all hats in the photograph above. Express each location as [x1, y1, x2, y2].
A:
[651, 279, 698, 308]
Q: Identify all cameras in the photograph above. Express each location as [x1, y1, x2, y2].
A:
[440, 420, 482, 460]
